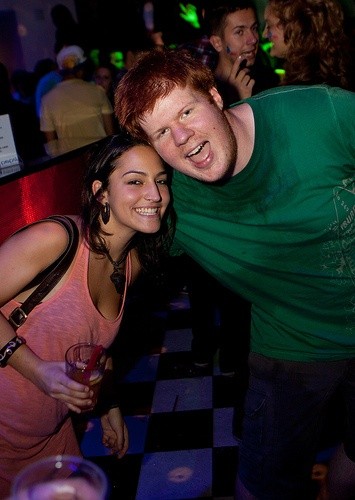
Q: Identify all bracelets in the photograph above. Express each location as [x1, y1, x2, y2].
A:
[0, 336, 27, 369]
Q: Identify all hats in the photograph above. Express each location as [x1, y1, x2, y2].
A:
[57, 46, 87, 70]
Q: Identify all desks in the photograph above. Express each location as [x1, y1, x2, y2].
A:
[0, 135, 116, 246]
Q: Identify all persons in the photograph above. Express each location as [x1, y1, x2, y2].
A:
[113, 47, 355, 500]
[189, 0, 289, 376]
[0, 0, 227, 159]
[0, 133, 179, 500]
[261, 0, 355, 90]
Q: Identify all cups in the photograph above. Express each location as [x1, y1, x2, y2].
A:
[12, 457, 108, 500]
[65, 343, 106, 412]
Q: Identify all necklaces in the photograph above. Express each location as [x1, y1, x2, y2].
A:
[94, 226, 131, 295]
